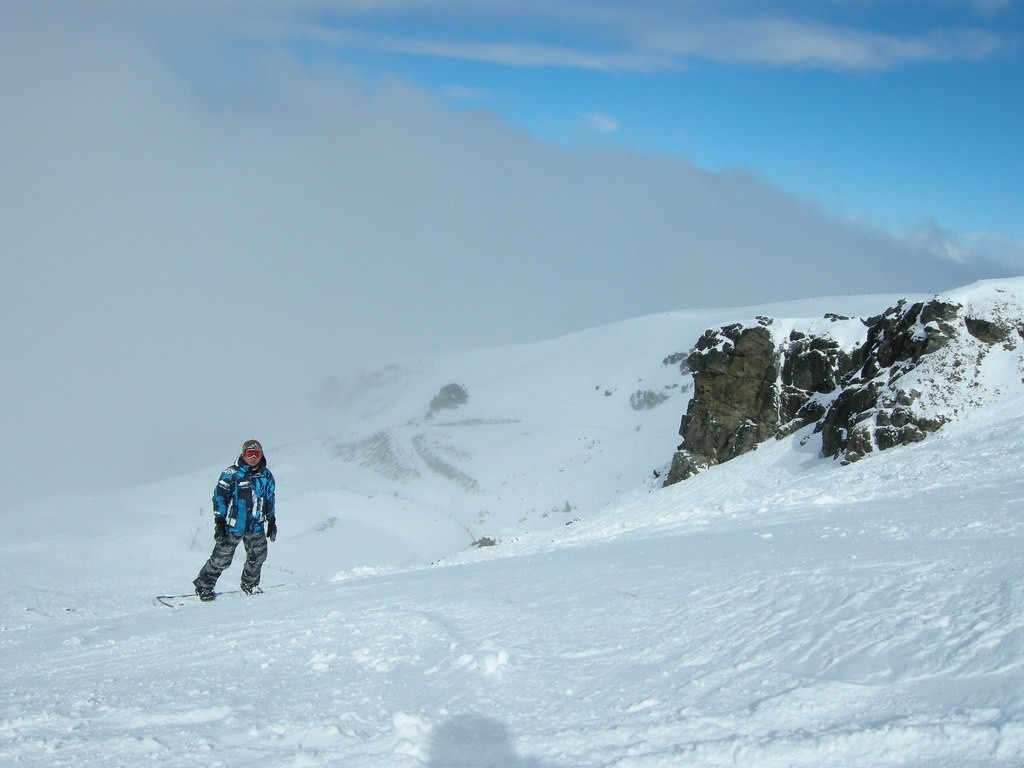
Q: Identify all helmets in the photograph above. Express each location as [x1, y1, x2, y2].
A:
[243, 440, 262, 455]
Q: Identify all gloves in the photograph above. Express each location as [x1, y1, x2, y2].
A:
[266, 519, 277, 542]
[214, 517, 228, 546]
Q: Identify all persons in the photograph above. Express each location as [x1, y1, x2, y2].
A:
[192, 440, 278, 602]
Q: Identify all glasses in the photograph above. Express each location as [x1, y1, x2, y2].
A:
[243, 449, 263, 459]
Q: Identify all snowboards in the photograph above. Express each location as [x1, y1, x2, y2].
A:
[154, 583, 288, 608]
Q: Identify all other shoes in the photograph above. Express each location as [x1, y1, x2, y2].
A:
[196, 586, 214, 601]
[240, 582, 263, 596]
